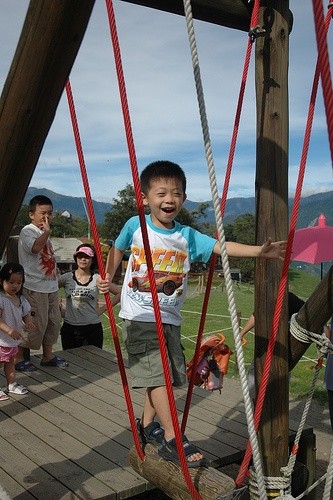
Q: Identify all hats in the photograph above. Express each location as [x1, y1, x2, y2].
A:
[75, 247, 95, 257]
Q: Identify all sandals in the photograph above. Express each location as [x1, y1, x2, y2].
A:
[0, 388, 9, 400]
[158, 434, 208, 467]
[6, 382, 29, 394]
[137, 422, 165, 443]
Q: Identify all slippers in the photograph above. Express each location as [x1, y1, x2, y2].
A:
[14, 360, 37, 371]
[40, 356, 69, 367]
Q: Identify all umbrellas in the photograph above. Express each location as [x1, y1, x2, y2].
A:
[290, 225, 333, 280]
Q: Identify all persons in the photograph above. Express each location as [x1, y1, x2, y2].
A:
[0, 262, 35, 401]
[97, 161, 287, 467]
[15, 195, 69, 371]
[323, 317, 333, 431]
[58, 243, 121, 351]
